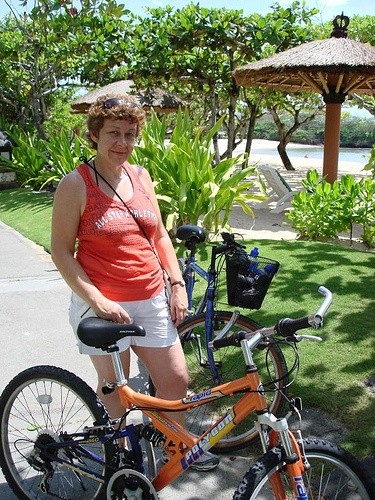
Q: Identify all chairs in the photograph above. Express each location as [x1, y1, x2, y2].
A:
[255, 167, 301, 214]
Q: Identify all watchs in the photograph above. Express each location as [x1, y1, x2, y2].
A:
[171, 280, 186, 287]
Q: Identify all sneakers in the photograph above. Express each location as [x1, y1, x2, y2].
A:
[160, 447, 219, 472]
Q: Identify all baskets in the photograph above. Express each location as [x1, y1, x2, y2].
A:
[226, 254, 281, 310]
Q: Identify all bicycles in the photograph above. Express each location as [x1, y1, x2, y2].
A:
[147, 225, 291, 455]
[0, 285, 375, 500]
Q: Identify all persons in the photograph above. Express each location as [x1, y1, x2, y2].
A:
[50, 94, 220, 472]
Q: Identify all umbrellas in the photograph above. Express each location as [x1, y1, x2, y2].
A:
[231, 12, 375, 185]
[69, 69, 200, 115]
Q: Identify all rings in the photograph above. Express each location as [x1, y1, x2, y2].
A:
[112, 320, 117, 323]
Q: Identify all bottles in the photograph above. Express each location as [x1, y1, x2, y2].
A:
[247, 247, 259, 283]
[253, 263, 273, 281]
